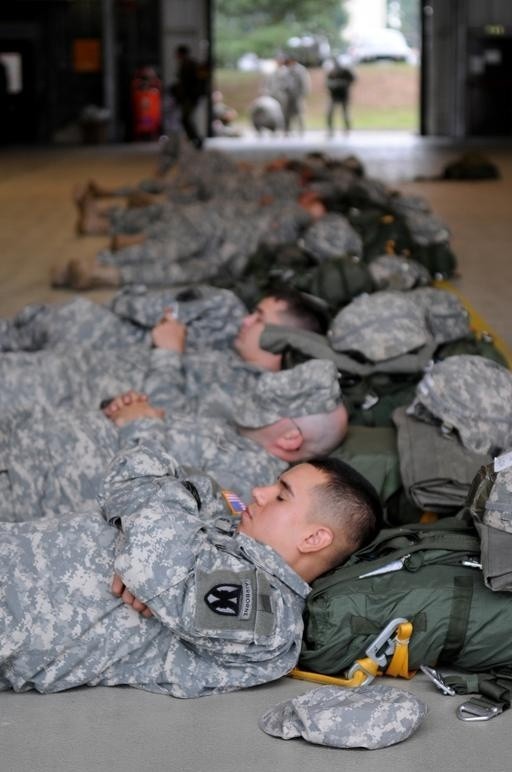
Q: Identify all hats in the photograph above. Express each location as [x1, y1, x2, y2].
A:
[414, 354, 512, 455]
[482, 466, 512, 533]
[287, 154, 470, 361]
[258, 684, 427, 750]
[234, 359, 343, 427]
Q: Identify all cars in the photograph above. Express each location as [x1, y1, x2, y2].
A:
[348, 26, 411, 65]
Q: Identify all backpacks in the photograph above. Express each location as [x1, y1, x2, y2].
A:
[339, 371, 422, 427]
[327, 425, 422, 528]
[302, 516, 512, 722]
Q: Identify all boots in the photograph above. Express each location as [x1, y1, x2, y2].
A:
[49, 177, 167, 289]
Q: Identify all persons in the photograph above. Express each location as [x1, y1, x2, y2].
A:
[1, 358, 348, 526]
[0, 444, 384, 700]
[162, 43, 355, 150]
[49, 154, 347, 290]
[1, 274, 271, 352]
[1, 288, 331, 429]
